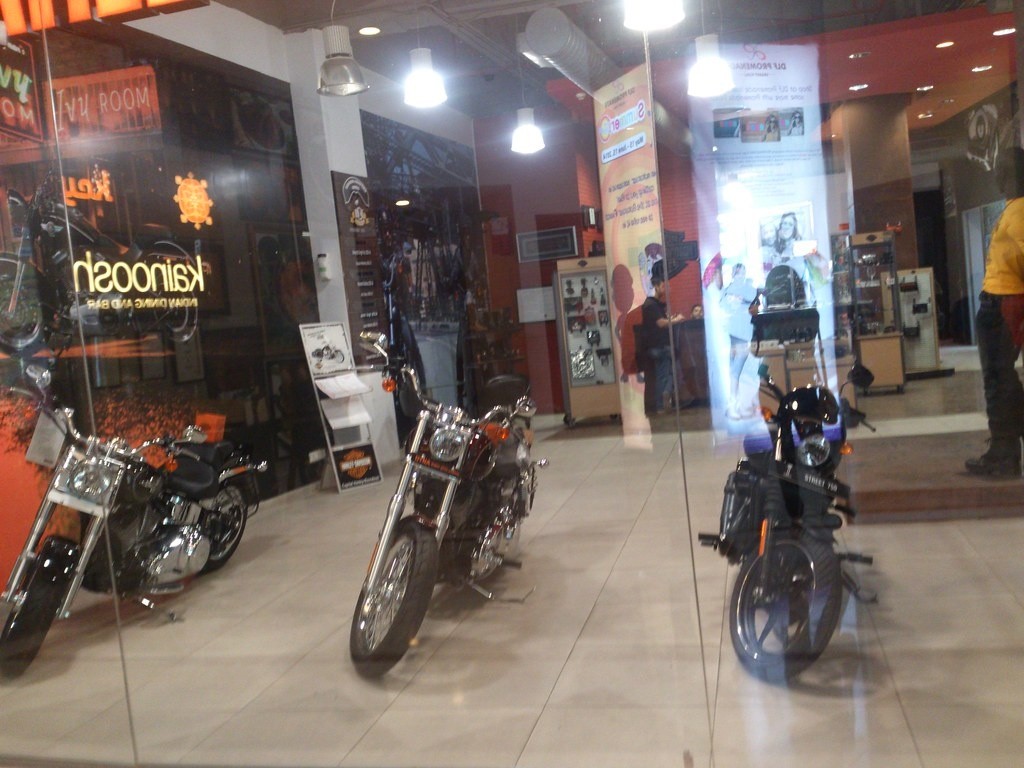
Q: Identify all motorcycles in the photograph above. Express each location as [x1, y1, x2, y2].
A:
[697, 360, 877, 688]
[351, 327, 550, 679]
[3, 366, 267, 675]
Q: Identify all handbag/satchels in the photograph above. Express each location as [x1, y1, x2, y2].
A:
[749, 265, 807, 315]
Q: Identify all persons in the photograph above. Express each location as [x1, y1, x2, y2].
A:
[787, 111, 804, 135]
[734, 108, 750, 138]
[690, 305, 704, 318]
[762, 213, 830, 311]
[703, 263, 762, 420]
[759, 114, 781, 142]
[966, 146, 1024, 477]
[642, 276, 686, 413]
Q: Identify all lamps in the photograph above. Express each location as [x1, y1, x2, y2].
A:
[403, 0, 448, 108]
[510, 0, 546, 154]
[687, 0, 735, 97]
[315, 0, 370, 97]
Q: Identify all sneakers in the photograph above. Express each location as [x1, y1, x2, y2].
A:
[965, 451, 1022, 479]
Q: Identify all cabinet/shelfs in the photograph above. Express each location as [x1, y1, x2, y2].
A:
[848, 230, 906, 396]
